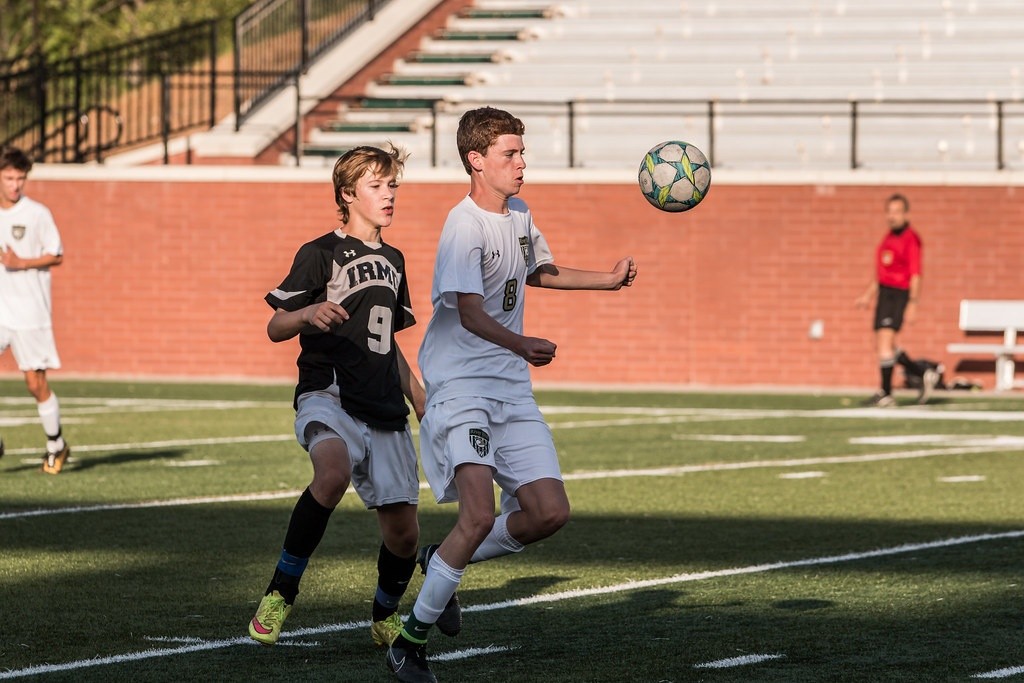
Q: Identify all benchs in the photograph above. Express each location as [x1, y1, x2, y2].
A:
[946, 299, 1023, 392]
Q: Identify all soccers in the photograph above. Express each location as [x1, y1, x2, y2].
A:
[637, 139, 713, 215]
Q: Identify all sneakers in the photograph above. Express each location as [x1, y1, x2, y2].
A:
[43, 443, 69, 474]
[417, 543, 462, 637]
[370, 611, 406, 647]
[385, 643, 436, 683]
[249, 590, 293, 645]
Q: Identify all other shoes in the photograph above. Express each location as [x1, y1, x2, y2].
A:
[859, 393, 894, 407]
[916, 368, 940, 405]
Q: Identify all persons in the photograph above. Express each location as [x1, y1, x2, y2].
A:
[382, 109, 638, 683]
[248, 139, 431, 649]
[0, 141, 72, 477]
[861, 191, 944, 407]
